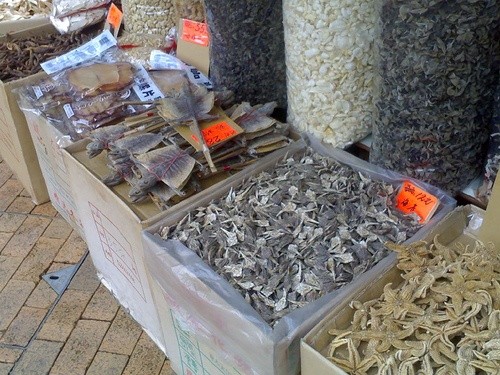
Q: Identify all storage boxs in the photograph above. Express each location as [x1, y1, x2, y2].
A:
[9, 46, 210, 248]
[0, 0, 55, 35]
[140, 133, 458, 375]
[297, 204, 500, 375]
[59, 87, 309, 360]
[0, 23, 133, 206]
